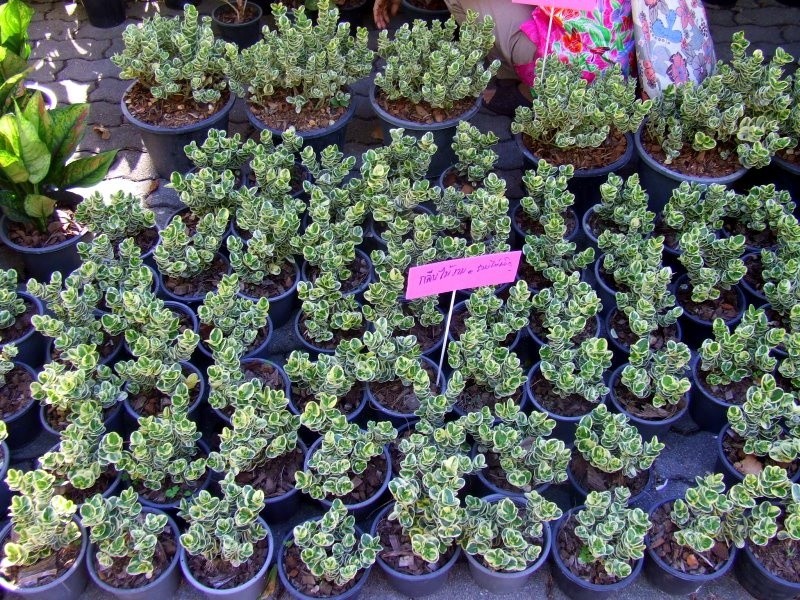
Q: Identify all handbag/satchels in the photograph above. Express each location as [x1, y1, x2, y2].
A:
[631, 0, 717, 101]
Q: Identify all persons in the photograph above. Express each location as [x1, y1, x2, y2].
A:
[372, 0, 635, 114]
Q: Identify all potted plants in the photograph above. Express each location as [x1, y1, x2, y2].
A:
[0, 0, 800, 600]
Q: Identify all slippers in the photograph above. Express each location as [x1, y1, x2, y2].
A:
[480, 85, 534, 119]
